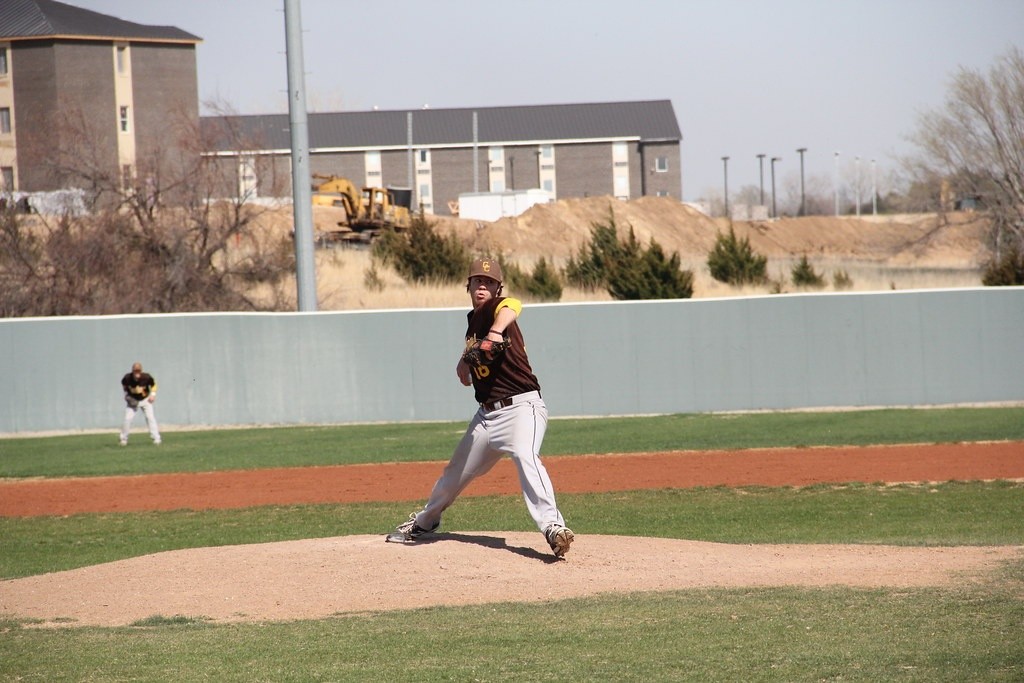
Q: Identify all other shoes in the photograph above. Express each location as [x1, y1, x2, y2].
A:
[153, 436, 162, 445]
[119, 439, 128, 446]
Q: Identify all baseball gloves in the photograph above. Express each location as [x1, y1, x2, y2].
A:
[125, 395, 138, 412]
[463, 336, 512, 386]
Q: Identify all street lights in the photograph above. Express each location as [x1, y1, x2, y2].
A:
[833, 152, 839, 217]
[871, 159, 877, 215]
[721, 156, 730, 216]
[796, 148, 806, 216]
[755, 154, 766, 205]
[855, 156, 861, 216]
[770, 157, 777, 218]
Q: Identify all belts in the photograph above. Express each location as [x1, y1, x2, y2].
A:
[481, 391, 542, 413]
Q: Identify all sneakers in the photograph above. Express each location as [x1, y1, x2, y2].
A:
[545, 524, 574, 556]
[393, 512, 440, 539]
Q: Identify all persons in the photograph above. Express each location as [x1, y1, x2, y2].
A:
[118, 363, 162, 447]
[387, 256, 575, 558]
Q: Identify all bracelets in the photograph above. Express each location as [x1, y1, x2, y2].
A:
[489, 329, 503, 335]
[152, 392, 155, 395]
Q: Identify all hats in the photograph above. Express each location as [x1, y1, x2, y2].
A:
[467, 257, 502, 286]
[132, 363, 143, 371]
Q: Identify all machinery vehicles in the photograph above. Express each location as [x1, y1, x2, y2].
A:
[311, 173, 414, 245]
[940, 181, 988, 213]
